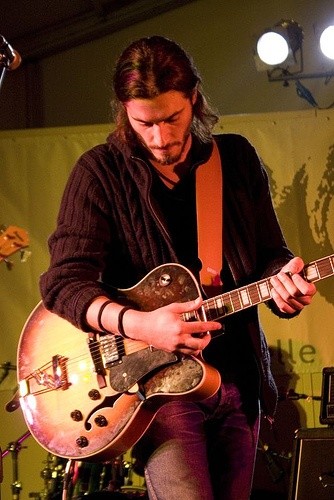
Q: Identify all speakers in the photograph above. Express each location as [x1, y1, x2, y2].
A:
[288, 427, 334, 500]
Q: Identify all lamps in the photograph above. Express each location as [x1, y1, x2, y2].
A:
[257, 22, 334, 82]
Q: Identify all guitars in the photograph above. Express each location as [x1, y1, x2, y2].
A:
[1, 226, 30, 269]
[15, 254, 334, 461]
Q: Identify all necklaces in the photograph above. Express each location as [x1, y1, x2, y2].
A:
[150, 161, 176, 186]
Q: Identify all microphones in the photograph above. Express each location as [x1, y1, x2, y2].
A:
[0, 34, 21, 72]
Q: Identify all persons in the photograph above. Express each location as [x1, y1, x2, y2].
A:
[39, 34, 315, 500]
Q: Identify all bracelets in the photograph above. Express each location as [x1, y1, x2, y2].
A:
[98, 300, 116, 335]
[118, 306, 136, 339]
